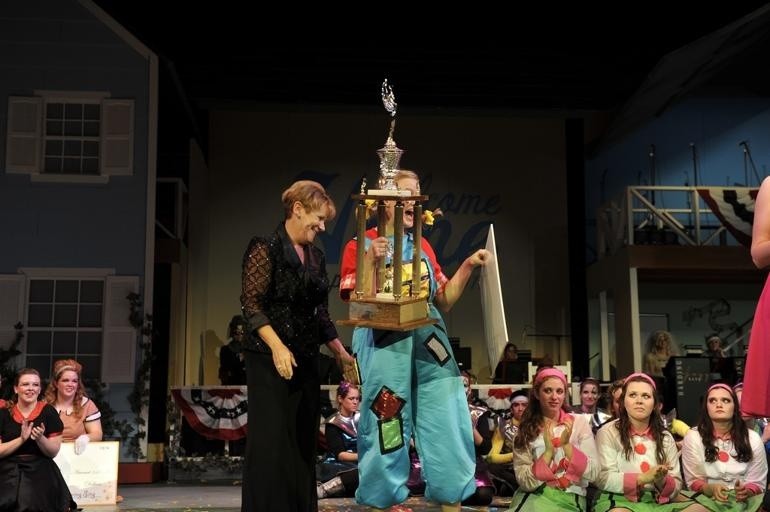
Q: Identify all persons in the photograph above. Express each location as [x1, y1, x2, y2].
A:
[242, 180, 355, 510]
[340, 171, 489, 511]
[316, 382, 363, 499]
[408, 331, 769, 512]
[42, 359, 104, 454]
[1, 368, 77, 511]
[740, 173, 770, 419]
[218, 314, 248, 385]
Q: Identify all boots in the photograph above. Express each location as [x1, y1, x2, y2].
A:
[317, 476, 346, 500]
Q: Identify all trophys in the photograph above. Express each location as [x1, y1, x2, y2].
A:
[367, 77, 412, 196]
